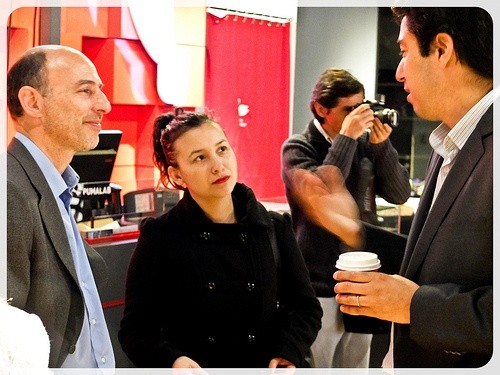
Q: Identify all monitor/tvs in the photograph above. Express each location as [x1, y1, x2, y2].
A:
[68, 130, 123, 223]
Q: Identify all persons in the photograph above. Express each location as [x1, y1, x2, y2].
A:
[333, 8, 494, 368]
[283, 71, 411, 368]
[8, 45, 117, 368]
[121, 109, 322, 369]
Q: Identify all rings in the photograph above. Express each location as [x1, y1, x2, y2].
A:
[356, 294, 360, 305]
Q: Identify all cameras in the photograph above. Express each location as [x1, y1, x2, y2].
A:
[358, 100, 400, 128]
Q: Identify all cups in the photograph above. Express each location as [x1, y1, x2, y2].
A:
[335, 252, 382, 333]
